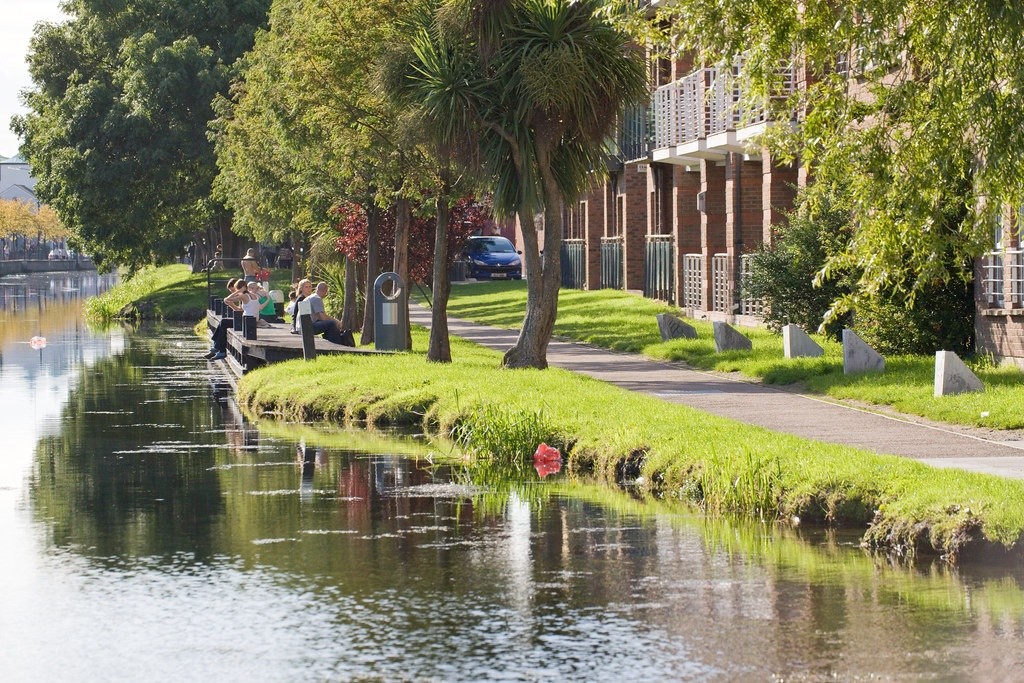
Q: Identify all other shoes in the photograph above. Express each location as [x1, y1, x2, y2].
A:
[211, 352, 226, 360]
[204, 349, 216, 358]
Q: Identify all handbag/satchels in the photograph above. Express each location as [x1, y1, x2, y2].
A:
[340, 329, 356, 347]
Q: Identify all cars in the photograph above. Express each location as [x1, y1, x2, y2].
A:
[459, 235, 522, 282]
[48, 248, 69, 259]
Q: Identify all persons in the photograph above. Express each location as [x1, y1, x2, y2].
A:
[487, 225, 500, 236]
[188, 241, 342, 361]
[241, 248, 258, 281]
